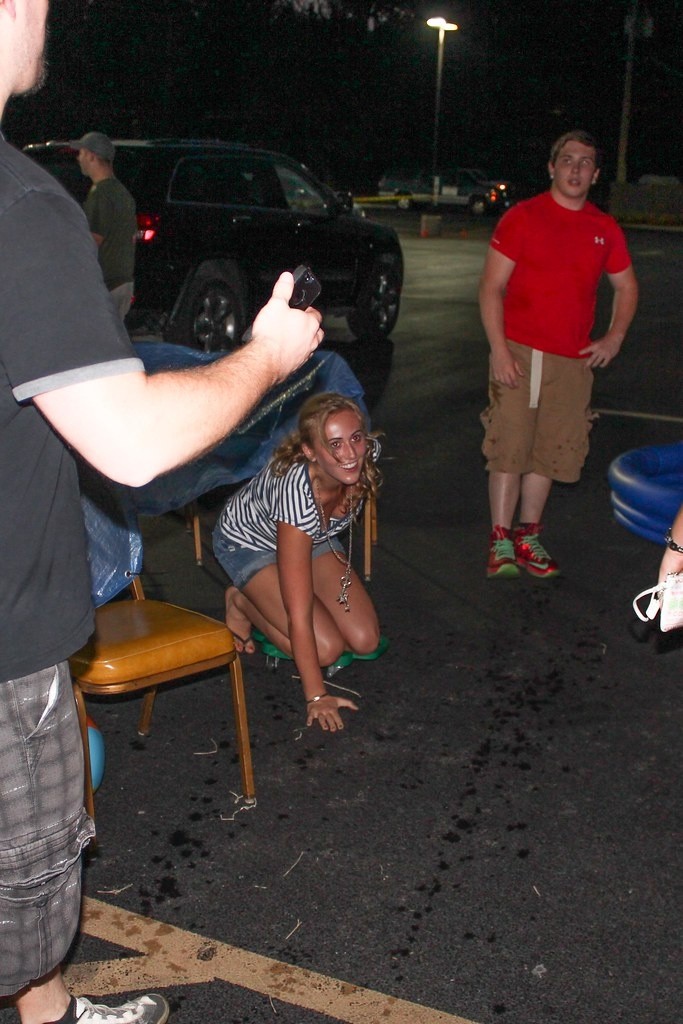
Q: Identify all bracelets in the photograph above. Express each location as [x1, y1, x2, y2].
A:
[664, 527, 683, 553]
[306, 693, 328, 704]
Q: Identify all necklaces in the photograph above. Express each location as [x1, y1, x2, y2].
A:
[314, 468, 354, 611]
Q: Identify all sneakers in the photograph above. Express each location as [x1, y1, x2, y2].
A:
[487, 523, 522, 581]
[69, 989, 169, 1023]
[513, 523, 562, 579]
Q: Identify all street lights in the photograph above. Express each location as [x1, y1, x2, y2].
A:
[425, 16, 459, 172]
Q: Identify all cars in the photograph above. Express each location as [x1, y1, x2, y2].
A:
[377, 161, 513, 220]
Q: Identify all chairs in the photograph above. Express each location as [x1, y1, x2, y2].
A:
[68, 577, 255, 827]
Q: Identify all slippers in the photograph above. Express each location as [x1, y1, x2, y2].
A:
[226, 622, 256, 656]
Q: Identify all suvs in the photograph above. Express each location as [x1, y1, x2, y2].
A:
[21, 134, 405, 355]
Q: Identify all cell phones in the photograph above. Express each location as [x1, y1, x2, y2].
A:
[242, 264, 322, 344]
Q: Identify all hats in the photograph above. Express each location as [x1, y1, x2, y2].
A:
[70, 132, 118, 161]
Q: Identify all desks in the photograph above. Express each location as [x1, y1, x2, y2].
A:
[80, 340, 384, 605]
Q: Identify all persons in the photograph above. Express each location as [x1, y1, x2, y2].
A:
[213, 393, 383, 731]
[657, 506, 683, 617]
[68, 132, 137, 325]
[0, 1, 325, 1024]
[479, 132, 637, 583]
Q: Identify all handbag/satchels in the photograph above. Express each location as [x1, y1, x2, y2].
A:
[633, 573, 683, 633]
